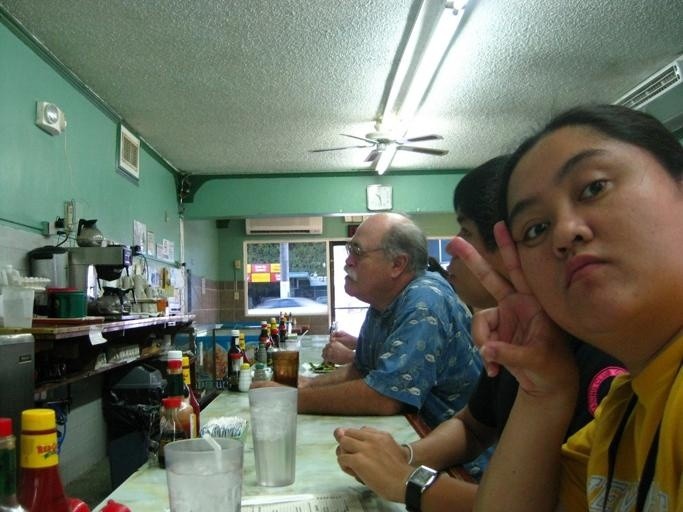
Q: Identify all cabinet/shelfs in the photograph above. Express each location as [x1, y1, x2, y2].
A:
[30, 312, 200, 403]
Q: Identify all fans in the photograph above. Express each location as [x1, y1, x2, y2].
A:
[306, 133, 449, 176]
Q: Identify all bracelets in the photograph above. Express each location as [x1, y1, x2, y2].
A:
[401, 440, 414, 466]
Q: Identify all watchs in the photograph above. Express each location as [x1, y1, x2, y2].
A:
[403, 464, 442, 512]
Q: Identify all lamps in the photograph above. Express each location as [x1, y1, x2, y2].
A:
[35, 102, 67, 135]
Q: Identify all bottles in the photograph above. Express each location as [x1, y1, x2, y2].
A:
[260, 324, 270, 348]
[239, 334, 250, 364]
[272, 311, 295, 347]
[21, 409, 90, 511]
[259, 344, 267, 367]
[180, 357, 199, 438]
[228, 331, 242, 393]
[158, 398, 187, 469]
[163, 360, 192, 440]
[0, 418, 25, 512]
[255, 364, 265, 382]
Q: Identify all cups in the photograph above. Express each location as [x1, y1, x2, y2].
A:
[248, 387, 298, 487]
[0, 288, 35, 328]
[163, 438, 244, 512]
[284, 339, 301, 351]
[272, 350, 299, 388]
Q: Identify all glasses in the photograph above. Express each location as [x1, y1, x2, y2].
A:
[345, 243, 385, 258]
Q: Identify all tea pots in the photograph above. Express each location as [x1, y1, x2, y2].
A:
[77, 218, 104, 247]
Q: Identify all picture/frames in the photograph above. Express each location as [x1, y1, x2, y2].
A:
[117, 125, 141, 181]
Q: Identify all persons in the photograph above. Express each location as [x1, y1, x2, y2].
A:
[322, 254, 448, 366]
[334, 149, 586, 511]
[448, 102, 682, 511]
[246, 211, 508, 481]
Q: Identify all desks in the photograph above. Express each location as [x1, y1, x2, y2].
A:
[88, 331, 461, 512]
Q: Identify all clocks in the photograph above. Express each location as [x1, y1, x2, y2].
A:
[366, 184, 392, 211]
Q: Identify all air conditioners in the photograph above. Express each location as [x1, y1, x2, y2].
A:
[243, 215, 323, 236]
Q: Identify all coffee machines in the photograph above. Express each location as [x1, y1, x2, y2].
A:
[26, 245, 132, 314]
